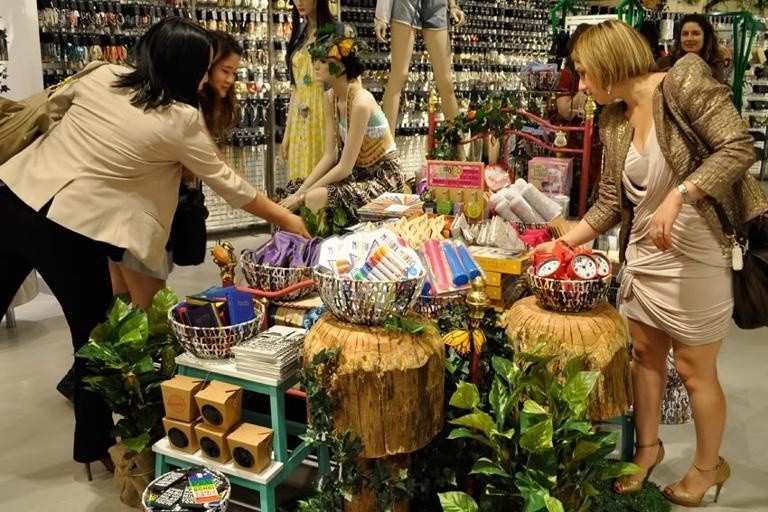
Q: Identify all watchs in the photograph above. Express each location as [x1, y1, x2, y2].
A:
[676, 184, 692, 205]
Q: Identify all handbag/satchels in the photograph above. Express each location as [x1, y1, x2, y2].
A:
[731, 215, 768, 329]
[1, 89, 50, 165]
[165, 183, 209, 266]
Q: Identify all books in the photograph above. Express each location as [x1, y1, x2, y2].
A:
[173, 281, 255, 336]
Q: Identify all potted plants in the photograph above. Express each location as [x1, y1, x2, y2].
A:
[430, 107, 487, 163]
[468, 89, 525, 165]
[74, 286, 184, 512]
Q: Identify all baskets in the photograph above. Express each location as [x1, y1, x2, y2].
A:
[142, 462, 232, 512]
[312, 264, 426, 325]
[168, 296, 266, 359]
[241, 250, 314, 302]
[517, 71, 562, 92]
[659, 352, 695, 426]
[416, 292, 470, 320]
[527, 265, 613, 314]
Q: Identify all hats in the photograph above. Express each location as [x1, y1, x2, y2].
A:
[305, 23, 366, 78]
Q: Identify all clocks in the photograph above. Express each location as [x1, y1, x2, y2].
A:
[530, 237, 612, 311]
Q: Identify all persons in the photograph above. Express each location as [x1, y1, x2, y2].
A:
[498, 18, 761, 507]
[651, 12, 727, 87]
[554, 21, 606, 217]
[274, 35, 403, 213]
[276, 0, 355, 182]
[1, 14, 317, 485]
[107, 32, 245, 307]
[367, 0, 466, 142]
[630, 19, 661, 65]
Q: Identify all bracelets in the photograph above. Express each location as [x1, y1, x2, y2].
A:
[449, 5, 457, 9]
[296, 192, 307, 205]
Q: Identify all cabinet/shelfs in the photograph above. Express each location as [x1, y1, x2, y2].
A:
[742, 77, 768, 119]
[151, 348, 330, 512]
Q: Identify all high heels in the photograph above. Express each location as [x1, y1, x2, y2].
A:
[612, 439, 664, 495]
[85, 457, 115, 481]
[661, 455, 730, 508]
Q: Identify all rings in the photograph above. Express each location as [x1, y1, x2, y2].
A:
[655, 230, 664, 239]
